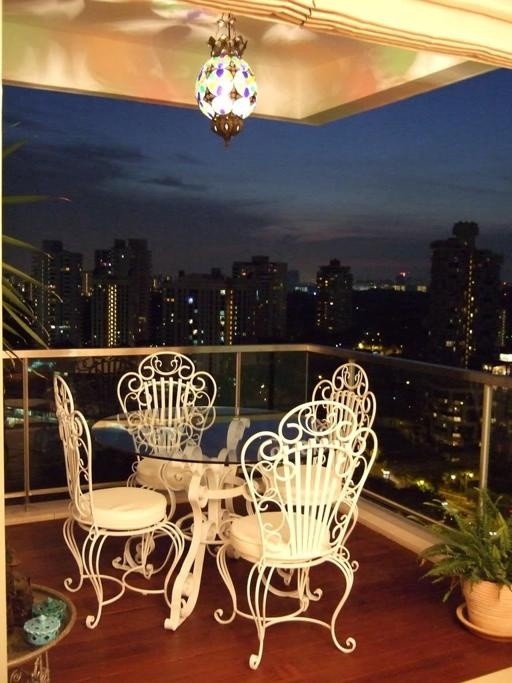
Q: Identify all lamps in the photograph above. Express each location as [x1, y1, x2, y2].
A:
[195, 9, 257, 150]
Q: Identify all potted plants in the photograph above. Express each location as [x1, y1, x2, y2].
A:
[406, 488, 511, 644]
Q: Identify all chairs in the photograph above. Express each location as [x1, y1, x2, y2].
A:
[53, 371, 185, 630]
[258, 361, 376, 588]
[214, 399, 378, 670]
[110, 351, 243, 575]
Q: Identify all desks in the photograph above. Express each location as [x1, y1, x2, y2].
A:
[7, 579, 78, 681]
[89, 404, 330, 626]
